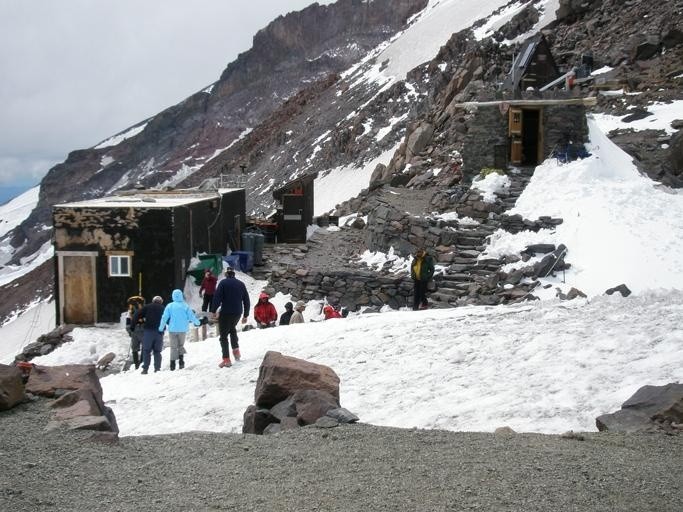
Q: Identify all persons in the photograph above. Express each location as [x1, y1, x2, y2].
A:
[125, 296, 146, 370]
[254, 293, 277, 329]
[333, 304, 341, 318]
[159, 289, 201, 371]
[199, 269, 218, 313]
[210, 267, 250, 368]
[130, 296, 170, 374]
[411, 247, 434, 310]
[324, 306, 339, 320]
[289, 301, 306, 325]
[342, 308, 349, 318]
[279, 302, 294, 325]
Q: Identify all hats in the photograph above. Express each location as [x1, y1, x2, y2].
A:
[225, 267, 235, 273]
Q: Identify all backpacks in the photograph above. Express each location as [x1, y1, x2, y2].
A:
[126, 296, 146, 328]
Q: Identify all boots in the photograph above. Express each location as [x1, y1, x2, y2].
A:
[218, 358, 231, 367]
[169, 360, 174, 370]
[132, 349, 143, 369]
[232, 348, 241, 358]
[153, 368, 160, 374]
[178, 355, 184, 369]
[140, 367, 149, 375]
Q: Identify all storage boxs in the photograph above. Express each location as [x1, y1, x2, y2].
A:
[186, 257, 216, 285]
[222, 254, 241, 273]
[229, 250, 253, 273]
[198, 253, 221, 275]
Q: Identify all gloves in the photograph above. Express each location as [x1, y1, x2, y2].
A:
[157, 327, 163, 336]
[198, 316, 209, 327]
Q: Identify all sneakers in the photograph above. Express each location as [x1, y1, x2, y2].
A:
[412, 305, 428, 311]
